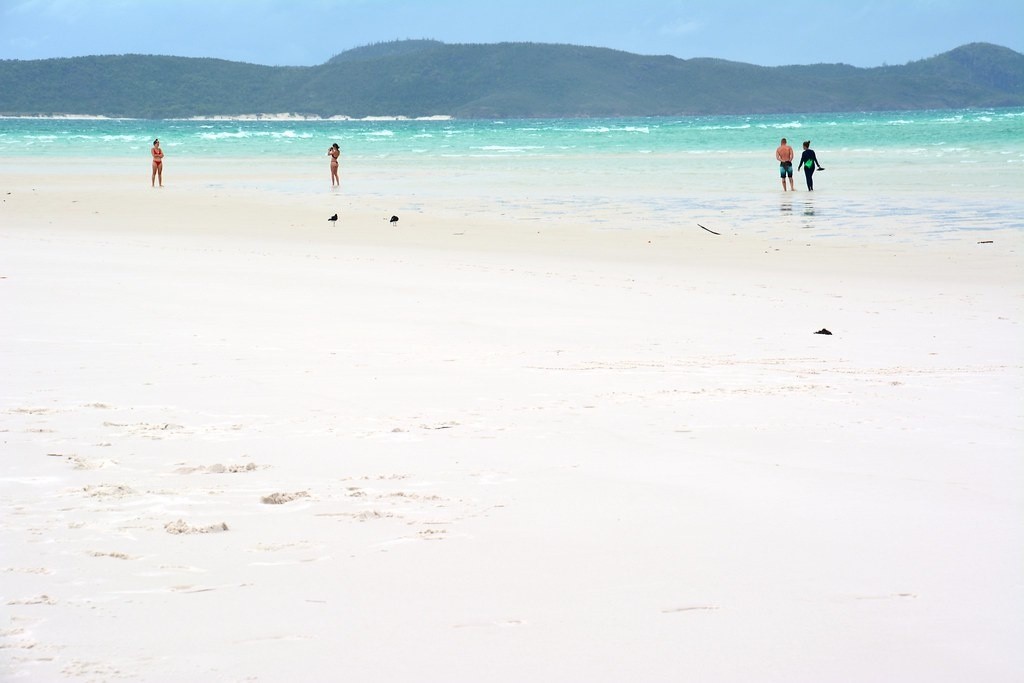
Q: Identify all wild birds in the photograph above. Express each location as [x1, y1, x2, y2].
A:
[328, 214, 338, 228]
[389, 215, 399, 226]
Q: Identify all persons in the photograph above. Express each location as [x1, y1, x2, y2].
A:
[797, 141, 820, 191]
[151, 139, 164, 187]
[775, 138, 795, 191]
[328, 143, 339, 186]
